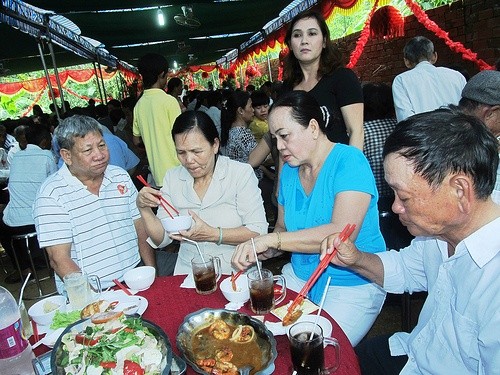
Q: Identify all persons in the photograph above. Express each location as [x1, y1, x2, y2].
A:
[453, 68, 500, 138]
[0, 78, 284, 284]
[137, 110, 269, 278]
[363, 84, 400, 234]
[317, 105, 500, 375]
[391, 36, 470, 124]
[230, 90, 390, 348]
[131, 56, 188, 187]
[250, 12, 366, 171]
[34, 117, 181, 293]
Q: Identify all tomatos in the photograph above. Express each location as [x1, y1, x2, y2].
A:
[111, 327, 134, 334]
[100, 362, 116, 369]
[123, 360, 145, 375]
[76, 332, 99, 346]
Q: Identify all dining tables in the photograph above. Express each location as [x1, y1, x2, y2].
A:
[28, 274, 361, 375]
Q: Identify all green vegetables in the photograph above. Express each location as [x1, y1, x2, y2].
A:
[50, 309, 82, 329]
[55, 314, 155, 375]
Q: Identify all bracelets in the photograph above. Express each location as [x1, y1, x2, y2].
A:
[216, 226, 223, 246]
[276, 230, 282, 251]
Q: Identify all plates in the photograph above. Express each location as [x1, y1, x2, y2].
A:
[50, 313, 172, 375]
[175, 307, 278, 375]
[81, 296, 148, 319]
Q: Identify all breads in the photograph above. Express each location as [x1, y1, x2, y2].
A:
[91, 311, 123, 323]
[80, 299, 105, 319]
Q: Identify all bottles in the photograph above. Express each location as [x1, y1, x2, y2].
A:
[0, 286, 35, 375]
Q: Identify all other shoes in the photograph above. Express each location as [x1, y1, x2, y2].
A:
[3, 271, 34, 284]
[30, 258, 51, 269]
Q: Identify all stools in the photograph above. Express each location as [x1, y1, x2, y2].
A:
[10, 231, 57, 300]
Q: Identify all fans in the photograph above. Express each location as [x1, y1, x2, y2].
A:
[174, 41, 197, 65]
[174, 6, 201, 29]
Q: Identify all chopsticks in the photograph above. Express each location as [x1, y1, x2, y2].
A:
[113, 278, 132, 296]
[137, 175, 179, 218]
[287, 224, 356, 313]
[32, 319, 39, 343]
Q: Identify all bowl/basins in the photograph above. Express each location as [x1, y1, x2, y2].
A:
[28, 296, 66, 325]
[123, 266, 155, 291]
[286, 315, 333, 348]
[161, 216, 192, 234]
[219, 275, 250, 303]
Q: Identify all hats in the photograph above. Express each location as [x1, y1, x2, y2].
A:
[462, 70, 500, 106]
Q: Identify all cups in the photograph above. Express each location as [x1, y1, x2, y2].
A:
[288, 321, 341, 375]
[247, 269, 286, 314]
[16, 300, 30, 340]
[63, 271, 101, 309]
[191, 254, 222, 295]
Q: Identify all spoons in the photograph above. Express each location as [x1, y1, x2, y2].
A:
[239, 365, 254, 375]
